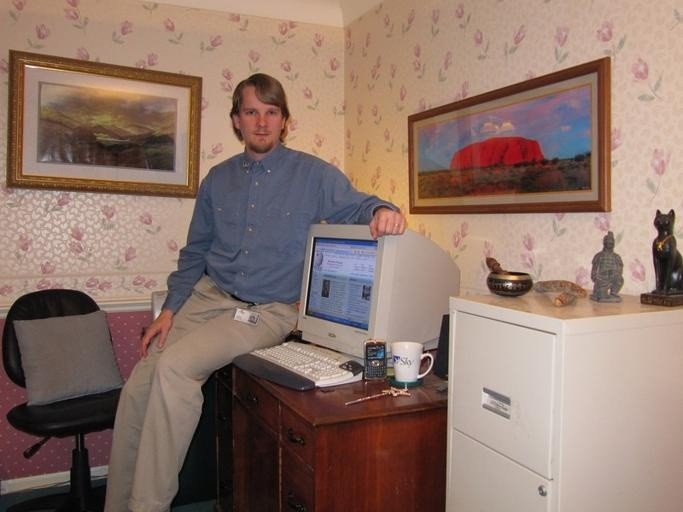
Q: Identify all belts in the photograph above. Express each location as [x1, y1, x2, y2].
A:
[205, 269, 255, 305]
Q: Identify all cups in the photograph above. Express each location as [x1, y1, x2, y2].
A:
[391, 341, 434, 382]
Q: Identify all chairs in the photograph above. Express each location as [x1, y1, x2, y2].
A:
[2, 288, 122, 512]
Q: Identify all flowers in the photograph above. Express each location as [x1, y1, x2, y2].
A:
[408, 56, 612, 215]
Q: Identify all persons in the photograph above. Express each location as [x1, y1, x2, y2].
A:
[101, 71, 407, 511]
[588, 230, 624, 302]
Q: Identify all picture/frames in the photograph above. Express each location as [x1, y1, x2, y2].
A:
[6, 49, 202, 199]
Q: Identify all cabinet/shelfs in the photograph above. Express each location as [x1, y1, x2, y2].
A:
[444, 289, 683, 511]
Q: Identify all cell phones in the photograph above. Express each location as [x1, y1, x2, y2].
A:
[365, 340, 387, 381]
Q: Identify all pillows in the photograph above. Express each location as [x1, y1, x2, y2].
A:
[12, 309, 124, 408]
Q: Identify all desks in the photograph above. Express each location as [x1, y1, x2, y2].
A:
[210, 349, 448, 512]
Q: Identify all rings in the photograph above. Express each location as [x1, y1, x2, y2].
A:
[393, 223, 400, 227]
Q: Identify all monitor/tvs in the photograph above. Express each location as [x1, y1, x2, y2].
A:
[297, 224, 460, 368]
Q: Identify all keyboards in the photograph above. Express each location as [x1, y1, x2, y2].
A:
[232, 342, 364, 391]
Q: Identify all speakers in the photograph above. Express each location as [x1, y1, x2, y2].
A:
[432, 314, 449, 378]
